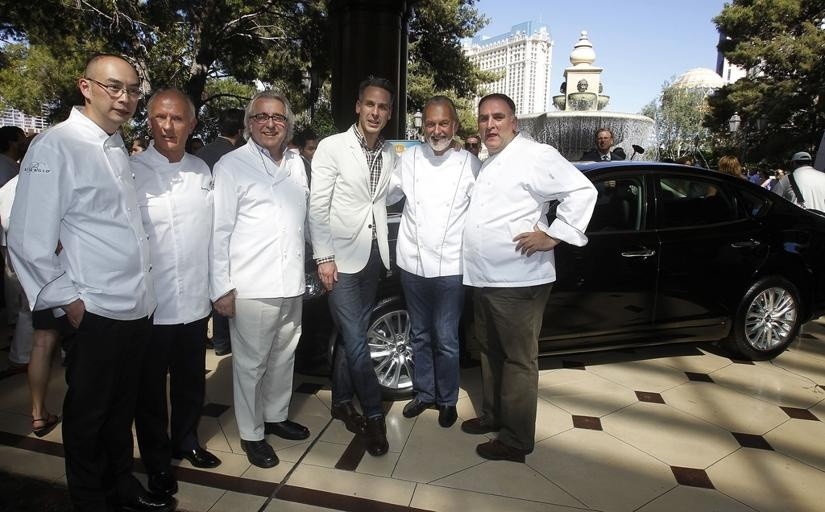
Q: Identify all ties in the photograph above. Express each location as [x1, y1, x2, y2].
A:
[603, 155, 607, 160]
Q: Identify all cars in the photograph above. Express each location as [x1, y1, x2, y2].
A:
[293, 160, 825, 400]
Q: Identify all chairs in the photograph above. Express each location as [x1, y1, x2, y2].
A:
[599, 192, 634, 232]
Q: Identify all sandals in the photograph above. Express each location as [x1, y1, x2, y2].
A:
[32, 412, 59, 437]
[7, 364, 28, 375]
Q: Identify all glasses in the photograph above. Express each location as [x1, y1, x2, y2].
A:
[465, 142, 478, 149]
[82, 76, 143, 104]
[249, 112, 289, 124]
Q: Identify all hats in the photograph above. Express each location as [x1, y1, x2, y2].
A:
[789, 152, 812, 167]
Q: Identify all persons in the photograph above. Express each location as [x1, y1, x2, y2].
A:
[2, 53, 598, 511]
[579, 127, 825, 218]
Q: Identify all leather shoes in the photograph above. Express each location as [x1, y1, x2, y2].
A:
[124, 489, 176, 511]
[241, 439, 279, 468]
[402, 397, 436, 418]
[264, 419, 310, 441]
[171, 448, 222, 468]
[365, 413, 389, 457]
[438, 406, 457, 428]
[205, 337, 231, 356]
[148, 465, 177, 496]
[330, 400, 369, 435]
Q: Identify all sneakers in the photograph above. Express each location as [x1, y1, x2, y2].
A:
[477, 440, 534, 461]
[461, 415, 499, 435]
